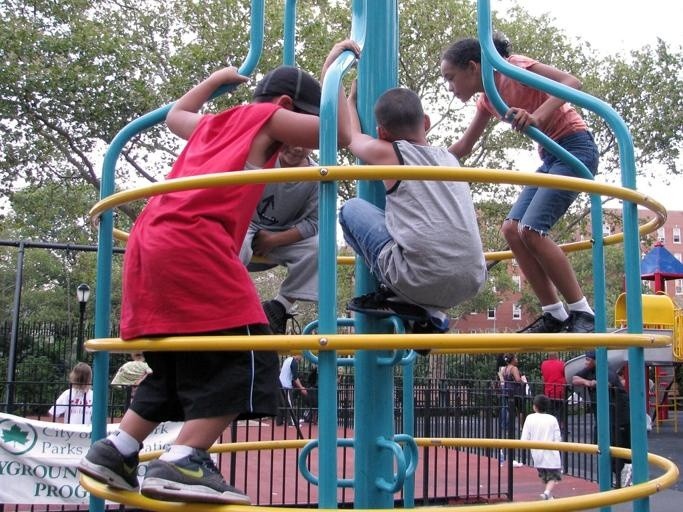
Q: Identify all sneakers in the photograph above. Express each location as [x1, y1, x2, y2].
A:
[517, 313, 570, 334]
[411, 310, 448, 356]
[143, 451, 253, 504]
[75, 440, 139, 491]
[347, 287, 428, 321]
[540, 492, 546, 502]
[513, 460, 524, 468]
[563, 311, 597, 334]
[259, 298, 291, 335]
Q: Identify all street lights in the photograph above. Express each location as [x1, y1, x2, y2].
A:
[75, 282, 90, 361]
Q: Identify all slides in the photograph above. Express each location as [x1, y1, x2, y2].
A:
[565, 329, 674, 403]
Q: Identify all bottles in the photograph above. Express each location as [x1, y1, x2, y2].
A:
[500, 449, 504, 467]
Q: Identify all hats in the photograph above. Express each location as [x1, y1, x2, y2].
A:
[586, 352, 596, 359]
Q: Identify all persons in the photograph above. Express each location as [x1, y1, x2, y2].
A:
[572, 349, 633, 489]
[540, 351, 571, 434]
[112, 352, 153, 388]
[76, 41, 364, 504]
[47, 361, 94, 424]
[496, 352, 530, 432]
[617, 370, 626, 386]
[239, 143, 319, 334]
[276, 350, 343, 429]
[439, 35, 599, 333]
[338, 79, 486, 357]
[520, 393, 562, 500]
